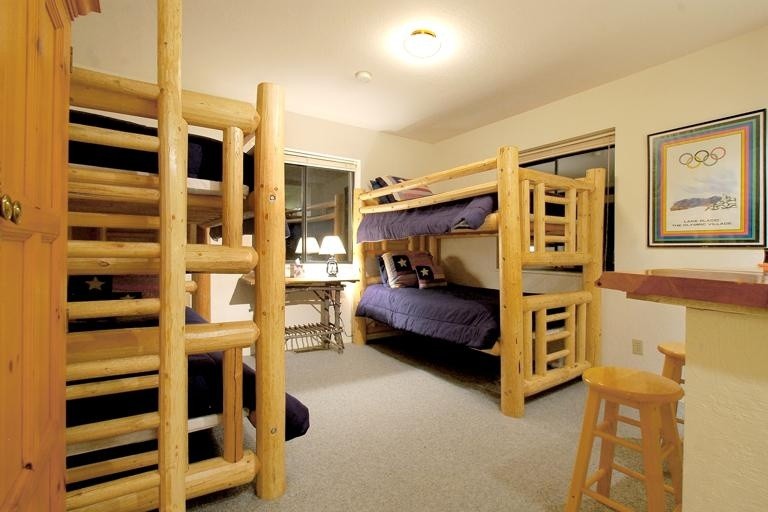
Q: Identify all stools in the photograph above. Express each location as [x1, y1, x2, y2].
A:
[563, 365, 682, 512]
[656, 342, 685, 430]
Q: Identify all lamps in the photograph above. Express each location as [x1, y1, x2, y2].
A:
[294, 235, 319, 260]
[401, 29, 442, 58]
[319, 235, 347, 276]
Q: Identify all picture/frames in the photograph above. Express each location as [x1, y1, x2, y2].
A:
[646, 108, 766, 249]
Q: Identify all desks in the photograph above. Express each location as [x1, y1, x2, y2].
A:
[245, 274, 361, 353]
[593, 270, 768, 512]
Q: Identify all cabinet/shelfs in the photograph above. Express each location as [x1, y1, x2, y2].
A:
[0, 0, 103, 511]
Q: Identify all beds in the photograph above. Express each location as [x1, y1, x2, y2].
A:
[352, 145, 608, 417]
[64, 63, 288, 511]
[285, 192, 348, 261]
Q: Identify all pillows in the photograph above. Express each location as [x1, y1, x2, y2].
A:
[376, 255, 390, 288]
[381, 247, 434, 289]
[413, 263, 448, 290]
[69, 275, 111, 304]
[110, 291, 142, 300]
[375, 174, 433, 204]
[369, 180, 390, 204]
